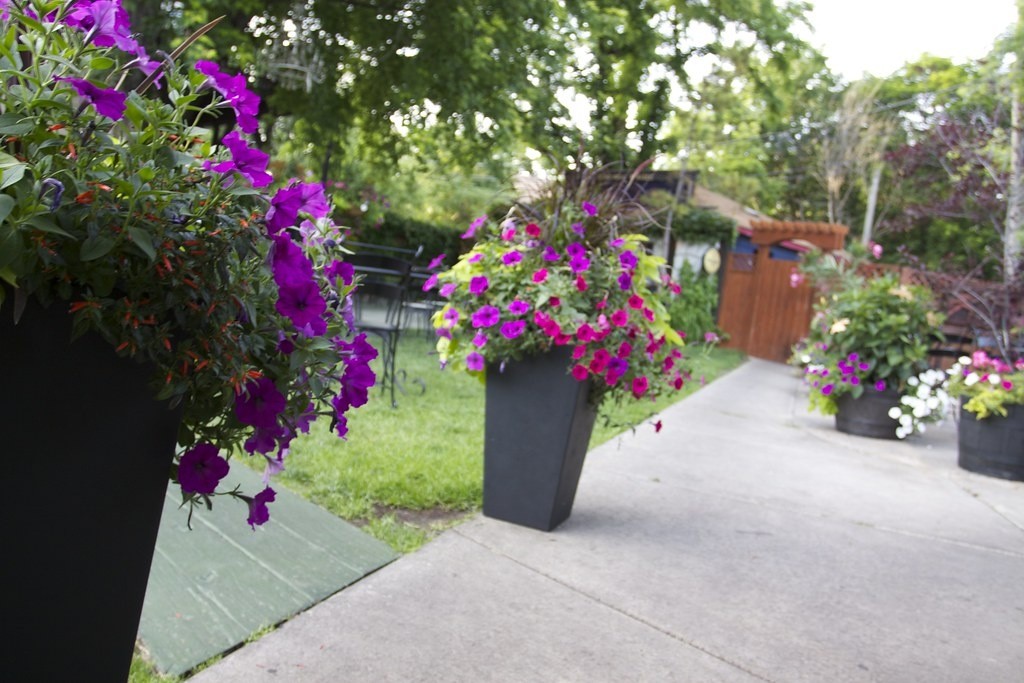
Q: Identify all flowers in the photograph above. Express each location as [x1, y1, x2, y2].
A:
[888, 353, 1024, 439]
[800, 270, 946, 416]
[423, 135, 719, 446]
[0, 0, 377, 529]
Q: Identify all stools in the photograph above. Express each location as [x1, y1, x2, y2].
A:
[428, 298, 448, 321]
[402, 301, 434, 338]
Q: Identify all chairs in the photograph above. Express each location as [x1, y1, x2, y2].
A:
[330, 242, 425, 411]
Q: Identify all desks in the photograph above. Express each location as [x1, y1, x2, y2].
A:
[346, 264, 431, 397]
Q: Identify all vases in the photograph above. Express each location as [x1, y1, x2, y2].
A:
[957, 395, 1024, 481]
[835, 386, 905, 441]
[0, 279, 197, 683]
[483, 338, 604, 532]
